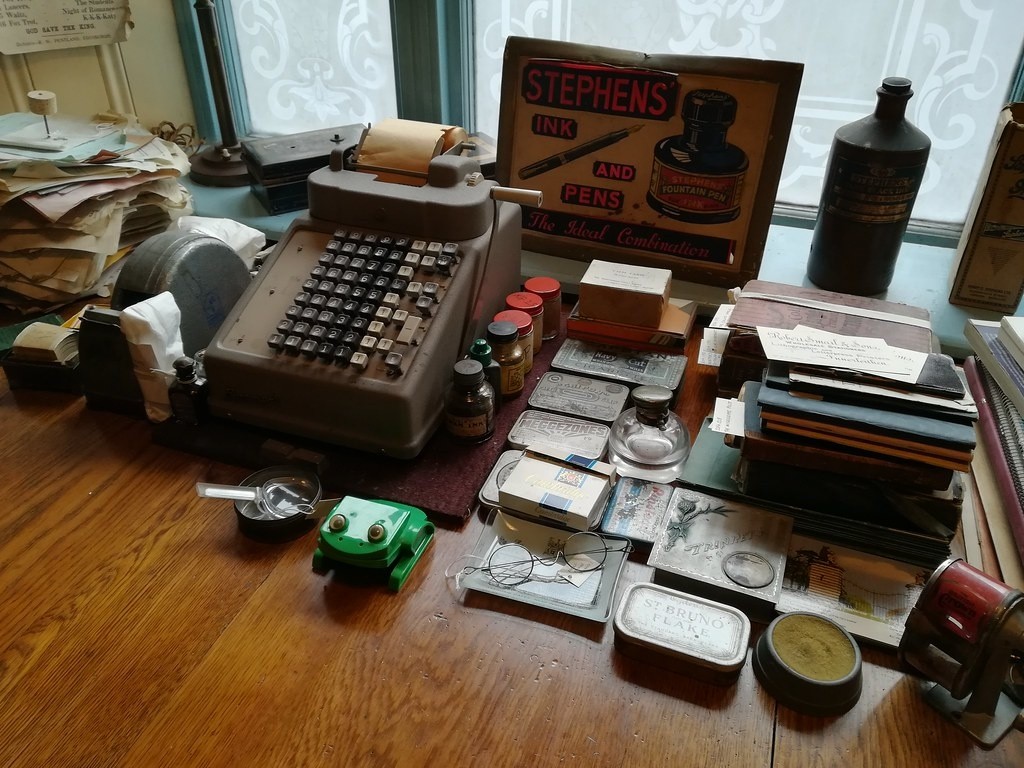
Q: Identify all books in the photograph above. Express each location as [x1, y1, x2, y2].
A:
[565, 295, 700, 357]
[672, 280, 1024, 593]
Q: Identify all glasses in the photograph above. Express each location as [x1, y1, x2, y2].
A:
[464, 531, 608, 586]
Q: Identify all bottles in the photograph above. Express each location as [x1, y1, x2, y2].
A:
[806, 76, 932, 296]
[608, 384, 691, 485]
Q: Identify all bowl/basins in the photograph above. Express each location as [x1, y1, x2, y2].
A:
[233, 465, 322, 543]
